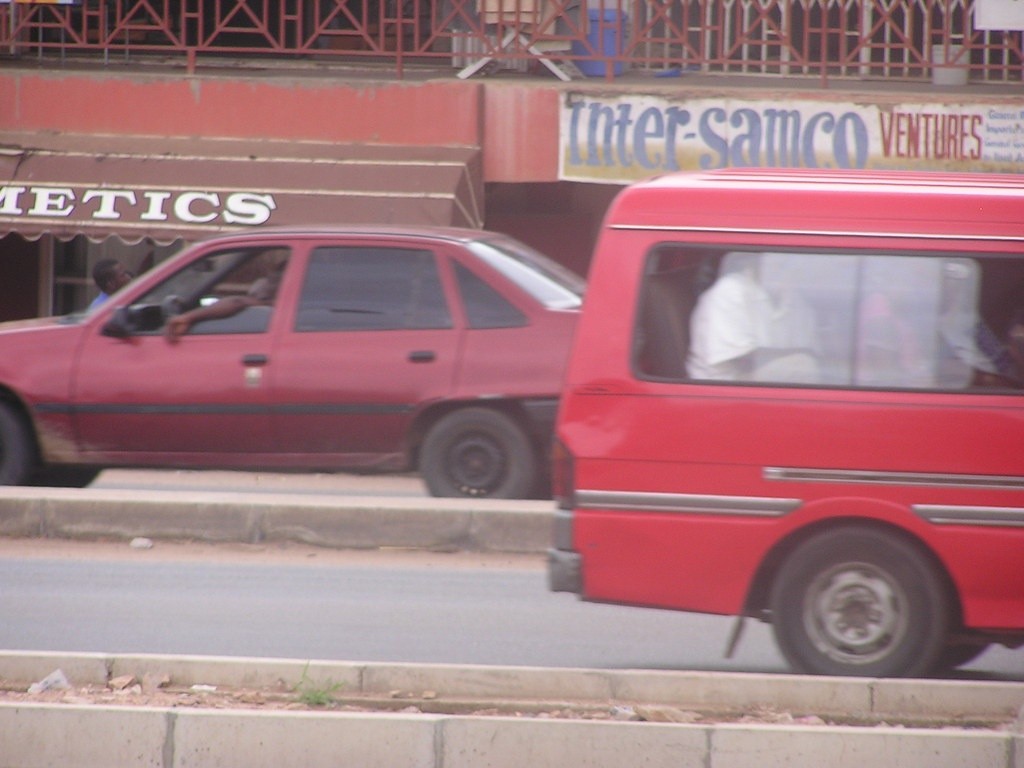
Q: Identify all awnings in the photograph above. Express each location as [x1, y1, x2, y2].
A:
[0, 130, 489, 247]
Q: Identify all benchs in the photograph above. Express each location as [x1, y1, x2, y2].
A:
[645, 264, 717, 383]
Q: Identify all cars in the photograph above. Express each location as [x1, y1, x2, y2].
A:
[1, 221, 587, 500]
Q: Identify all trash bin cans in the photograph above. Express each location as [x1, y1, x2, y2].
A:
[568, 10, 627, 77]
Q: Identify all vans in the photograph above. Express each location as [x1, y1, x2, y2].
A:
[544, 168, 1024, 676]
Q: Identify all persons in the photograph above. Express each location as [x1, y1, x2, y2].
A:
[683, 248, 1024, 393]
[87, 258, 132, 310]
[159, 259, 287, 346]
